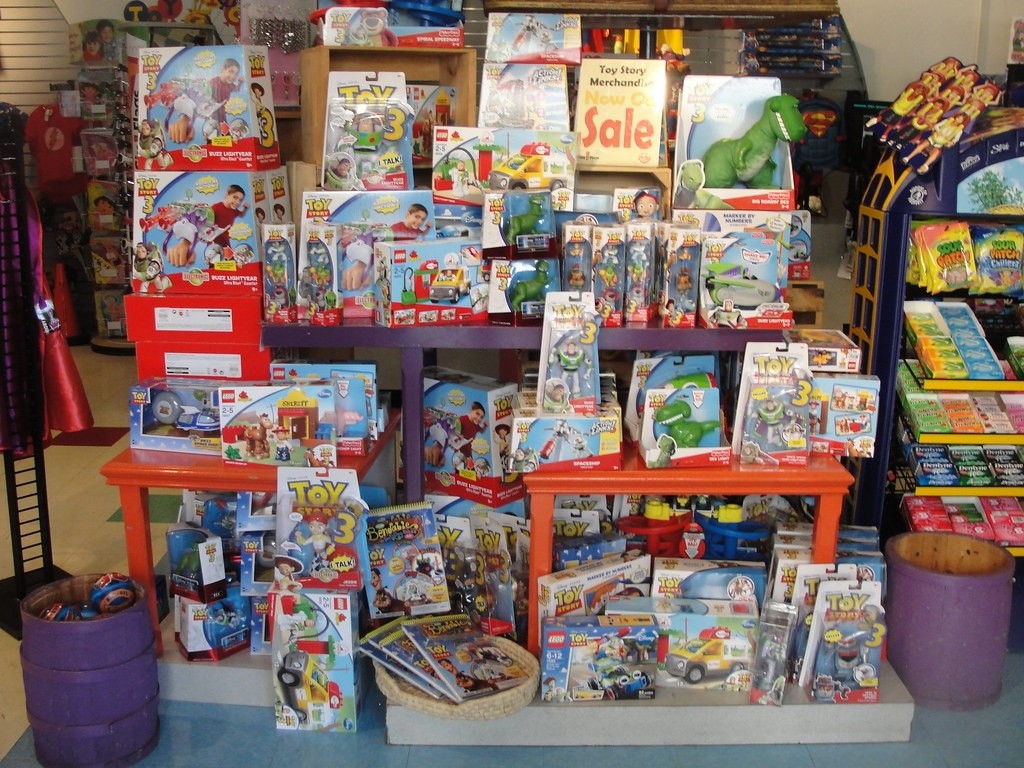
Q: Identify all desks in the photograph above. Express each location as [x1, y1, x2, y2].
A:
[99, 388, 405, 657]
[522, 446, 854, 657]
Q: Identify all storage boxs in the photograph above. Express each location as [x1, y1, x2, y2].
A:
[895, 301, 1024, 546]
[84, 0, 890, 732]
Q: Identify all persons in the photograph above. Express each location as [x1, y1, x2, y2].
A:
[82, 31, 104, 64]
[297, 509, 334, 574]
[633, 192, 661, 223]
[419, 400, 489, 473]
[95, 19, 119, 64]
[341, 202, 432, 291]
[865, 57, 1006, 178]
[167, 184, 250, 269]
[167, 57, 244, 146]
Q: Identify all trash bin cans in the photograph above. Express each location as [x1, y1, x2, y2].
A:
[18, 571, 161, 768]
[885, 530, 1015, 714]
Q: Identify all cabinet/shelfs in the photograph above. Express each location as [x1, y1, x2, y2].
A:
[63, 14, 216, 356]
[302, 44, 476, 191]
[260, 324, 786, 504]
[845, 57, 1024, 653]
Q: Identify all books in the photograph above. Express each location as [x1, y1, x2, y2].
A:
[60, 18, 128, 338]
